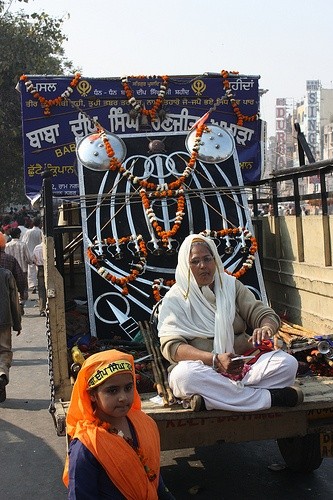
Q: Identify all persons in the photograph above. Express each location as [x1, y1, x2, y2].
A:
[158, 235, 304, 413]
[0, 206, 56, 402]
[248, 196, 333, 218]
[62, 349, 176, 500]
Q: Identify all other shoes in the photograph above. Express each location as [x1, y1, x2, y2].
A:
[0, 374, 7, 403]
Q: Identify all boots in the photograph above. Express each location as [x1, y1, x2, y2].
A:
[268, 386, 303, 403]
[191, 395, 204, 408]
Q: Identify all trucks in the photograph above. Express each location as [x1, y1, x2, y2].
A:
[42, 169, 332, 473]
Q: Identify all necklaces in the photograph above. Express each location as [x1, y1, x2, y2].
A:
[96, 419, 158, 481]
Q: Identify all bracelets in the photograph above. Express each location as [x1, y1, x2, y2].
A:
[211, 352, 218, 371]
